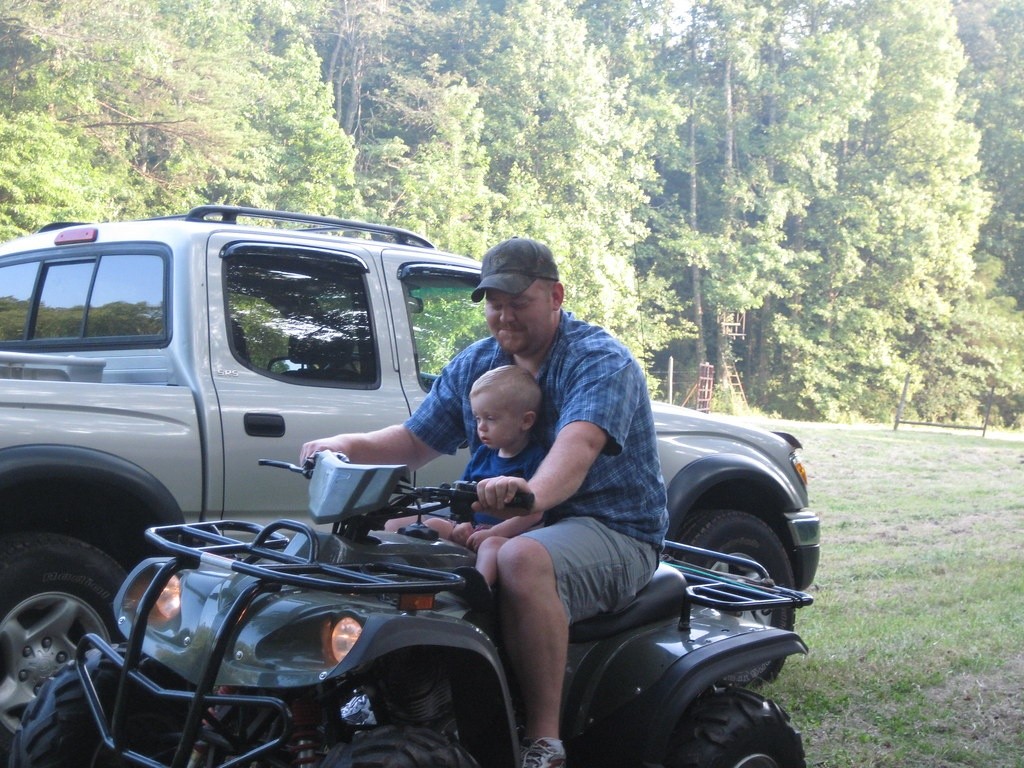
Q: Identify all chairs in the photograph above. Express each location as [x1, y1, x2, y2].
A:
[284, 323, 362, 382]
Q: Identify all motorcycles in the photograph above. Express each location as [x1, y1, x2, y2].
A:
[8, 449, 826, 768]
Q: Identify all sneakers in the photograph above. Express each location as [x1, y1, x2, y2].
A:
[521, 736, 566, 768]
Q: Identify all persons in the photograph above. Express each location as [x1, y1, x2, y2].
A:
[298, 236, 671, 766]
[425, 364, 547, 609]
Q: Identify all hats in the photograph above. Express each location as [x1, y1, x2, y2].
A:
[472, 236, 559, 303]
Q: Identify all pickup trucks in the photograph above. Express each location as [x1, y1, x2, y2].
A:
[0, 202, 819, 694]
